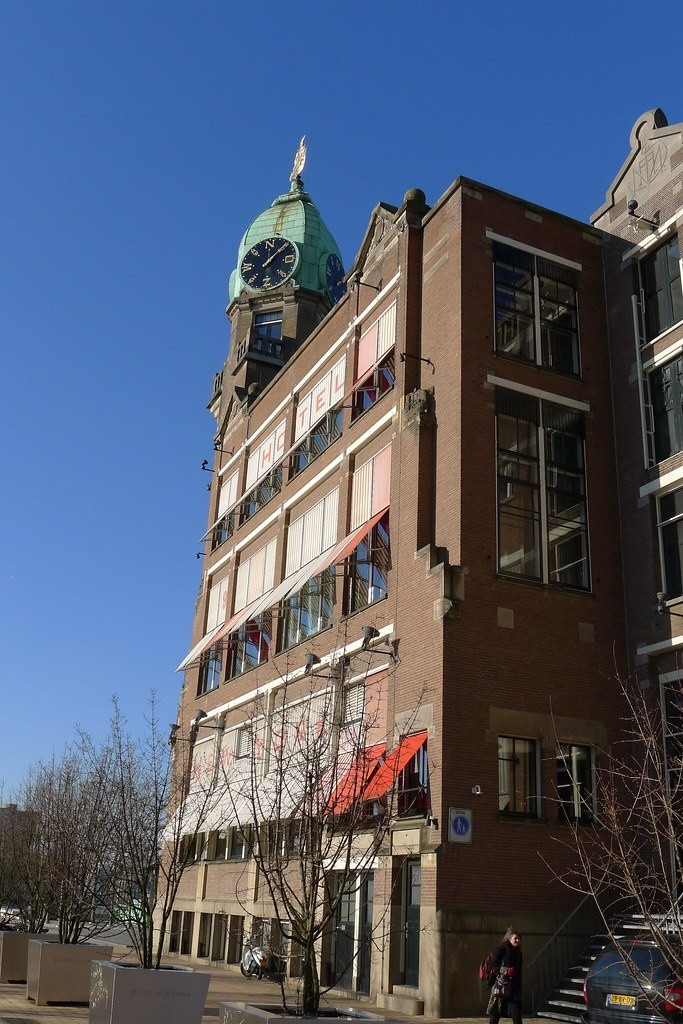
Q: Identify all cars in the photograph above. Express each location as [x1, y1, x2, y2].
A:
[582, 933, 683, 1024]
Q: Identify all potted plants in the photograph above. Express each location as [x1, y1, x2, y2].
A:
[0, 604, 439, 1024]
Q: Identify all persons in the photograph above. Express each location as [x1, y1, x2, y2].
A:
[479, 926, 523, 1024]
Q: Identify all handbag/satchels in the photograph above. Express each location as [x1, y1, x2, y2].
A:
[491, 980, 511, 997]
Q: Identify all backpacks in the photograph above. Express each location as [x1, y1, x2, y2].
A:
[479, 944, 508, 980]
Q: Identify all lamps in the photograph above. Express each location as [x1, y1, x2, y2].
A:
[304, 653, 341, 679]
[196, 553, 207, 559]
[201, 460, 215, 472]
[168, 724, 196, 747]
[360, 626, 394, 658]
[194, 709, 225, 731]
[213, 440, 232, 455]
[424, 809, 438, 827]
[399, 353, 430, 363]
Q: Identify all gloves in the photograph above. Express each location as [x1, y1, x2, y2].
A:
[507, 967, 516, 976]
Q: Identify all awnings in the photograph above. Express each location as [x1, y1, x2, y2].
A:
[156, 731, 428, 837]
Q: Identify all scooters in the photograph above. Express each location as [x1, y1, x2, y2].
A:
[240, 926, 271, 981]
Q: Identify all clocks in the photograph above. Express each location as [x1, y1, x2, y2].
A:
[240, 237, 297, 289]
[326, 254, 347, 304]
